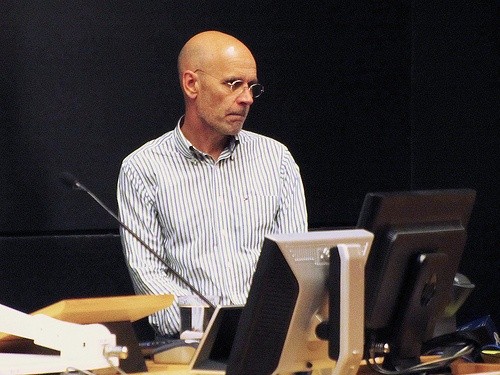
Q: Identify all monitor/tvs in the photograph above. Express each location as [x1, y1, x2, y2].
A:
[355, 188, 479, 355]
[186, 303, 246, 375]
[0, 295, 175, 353]
[222, 229, 375, 375]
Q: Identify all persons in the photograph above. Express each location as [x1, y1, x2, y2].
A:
[115, 31, 310, 342]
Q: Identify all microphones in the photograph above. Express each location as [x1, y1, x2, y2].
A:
[29, 168, 217, 311]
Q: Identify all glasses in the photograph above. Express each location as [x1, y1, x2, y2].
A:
[193, 69, 264, 98]
